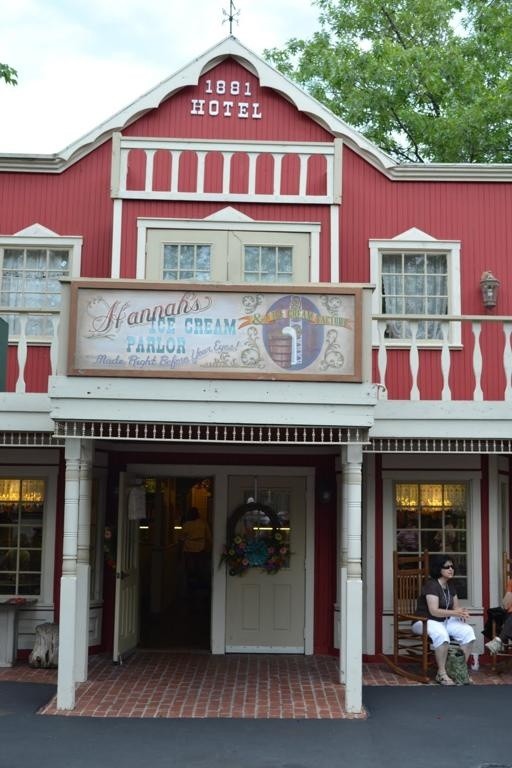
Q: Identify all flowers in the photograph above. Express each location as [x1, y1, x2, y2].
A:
[217, 501, 292, 578]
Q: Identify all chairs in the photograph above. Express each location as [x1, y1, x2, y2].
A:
[489, 551, 512, 669]
[377, 548, 478, 682]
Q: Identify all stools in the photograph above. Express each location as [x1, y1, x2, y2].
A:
[30, 622, 58, 669]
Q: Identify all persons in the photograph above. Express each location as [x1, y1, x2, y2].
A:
[485, 580, 512, 654]
[411, 555, 477, 686]
[433, 514, 458, 576]
[0, 535, 30, 567]
[175, 506, 212, 557]
[397, 521, 418, 552]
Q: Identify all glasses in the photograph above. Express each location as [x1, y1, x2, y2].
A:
[441, 564, 454, 569]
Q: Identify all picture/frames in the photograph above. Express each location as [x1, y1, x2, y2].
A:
[65, 278, 364, 384]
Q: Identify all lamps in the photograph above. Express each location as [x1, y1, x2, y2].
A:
[480, 270, 501, 308]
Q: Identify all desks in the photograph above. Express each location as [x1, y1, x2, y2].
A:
[0, 596, 39, 666]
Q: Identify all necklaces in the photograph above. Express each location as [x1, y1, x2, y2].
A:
[438, 578, 450, 621]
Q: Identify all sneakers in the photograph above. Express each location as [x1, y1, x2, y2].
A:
[435, 673, 455, 686]
[485, 637, 502, 655]
